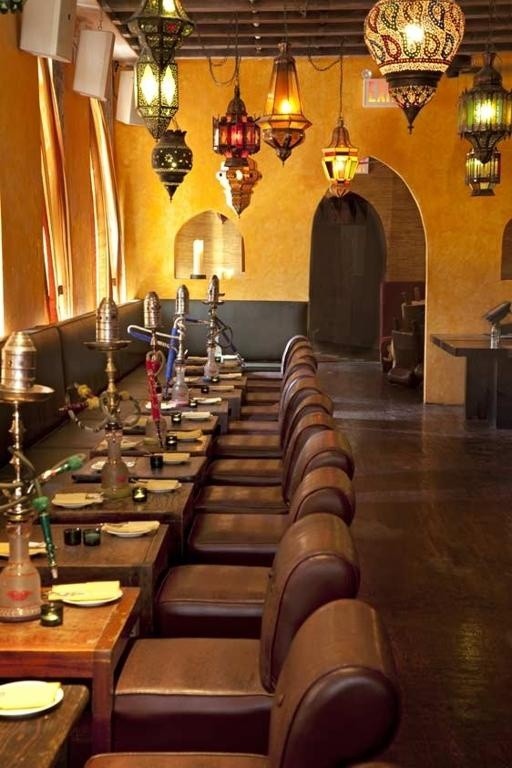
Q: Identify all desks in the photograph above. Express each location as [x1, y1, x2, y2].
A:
[431, 333, 512, 430]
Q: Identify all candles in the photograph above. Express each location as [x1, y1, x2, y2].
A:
[192, 239, 204, 275]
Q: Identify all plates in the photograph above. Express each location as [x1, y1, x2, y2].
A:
[137, 479, 184, 494]
[91, 417, 147, 472]
[144, 355, 242, 448]
[154, 451, 189, 464]
[63, 588, 125, 608]
[108, 522, 154, 538]
[1, 540, 48, 559]
[49, 494, 96, 509]
[0, 680, 64, 719]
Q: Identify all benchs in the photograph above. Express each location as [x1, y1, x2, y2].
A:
[0, 325, 69, 468]
[142, 298, 308, 373]
[53, 301, 146, 419]
[379, 280, 425, 373]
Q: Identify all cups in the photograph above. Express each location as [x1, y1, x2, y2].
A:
[41, 603, 65, 628]
[132, 484, 148, 502]
[151, 457, 164, 470]
[63, 527, 101, 546]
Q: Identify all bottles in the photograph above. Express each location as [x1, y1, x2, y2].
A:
[490, 323, 501, 349]
[95, 297, 121, 342]
[1, 523, 45, 623]
[1, 330, 38, 395]
[175, 284, 191, 315]
[142, 291, 163, 329]
[100, 425, 131, 500]
[206, 275, 220, 304]
[208, 336, 223, 359]
[202, 347, 219, 381]
